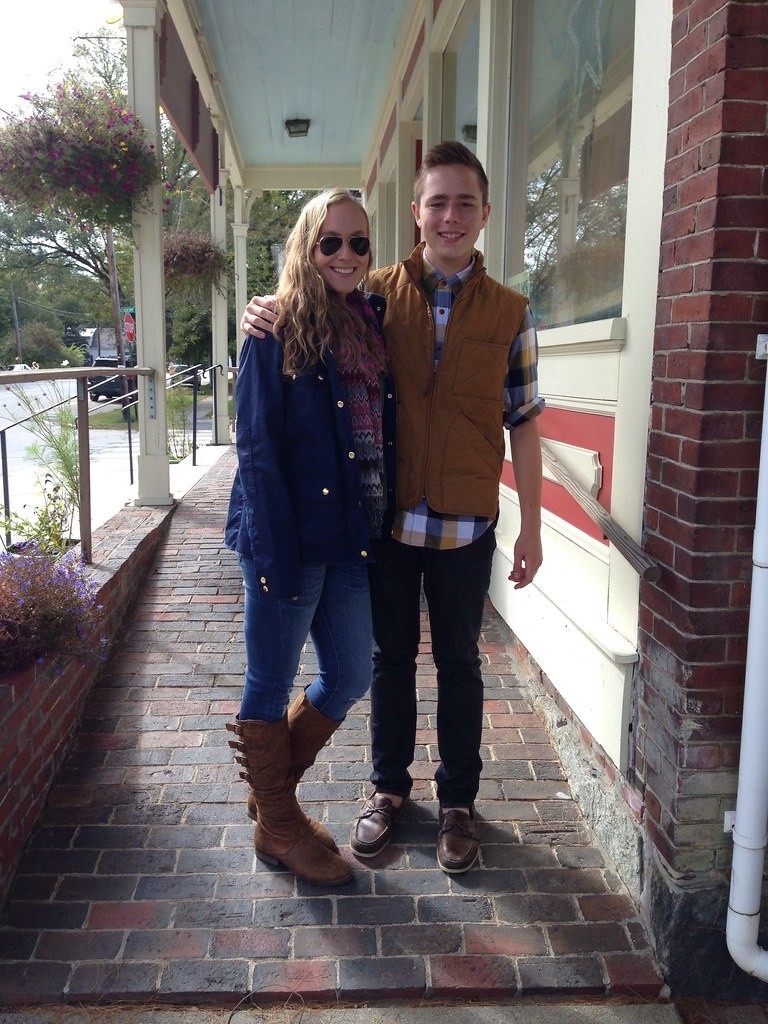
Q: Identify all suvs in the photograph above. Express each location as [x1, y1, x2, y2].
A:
[88, 357, 136, 401]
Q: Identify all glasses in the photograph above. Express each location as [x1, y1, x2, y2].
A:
[314, 235, 370, 257]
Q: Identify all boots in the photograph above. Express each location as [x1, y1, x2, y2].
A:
[226, 706, 354, 884]
[246, 685, 336, 853]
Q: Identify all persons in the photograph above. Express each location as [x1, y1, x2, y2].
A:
[240, 140, 546, 873]
[224, 186, 396, 887]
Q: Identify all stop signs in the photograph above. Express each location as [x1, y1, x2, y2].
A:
[124, 312, 135, 343]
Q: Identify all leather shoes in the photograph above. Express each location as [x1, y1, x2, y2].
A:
[436, 805, 481, 873]
[348, 789, 410, 858]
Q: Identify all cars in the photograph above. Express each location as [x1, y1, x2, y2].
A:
[0, 364, 33, 372]
[170, 365, 201, 387]
